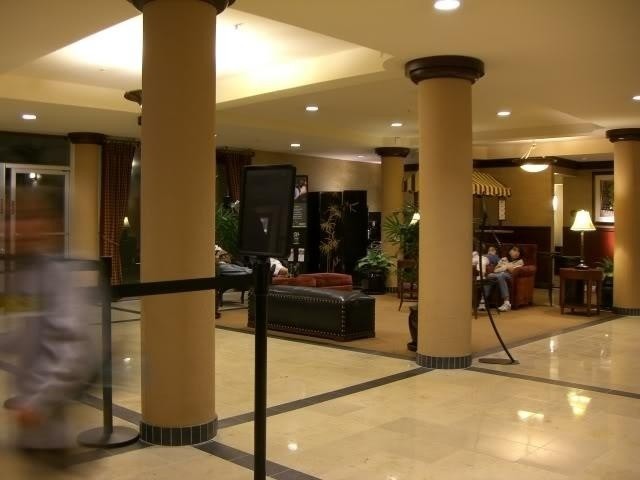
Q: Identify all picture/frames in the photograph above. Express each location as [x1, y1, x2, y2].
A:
[592, 171, 614, 226]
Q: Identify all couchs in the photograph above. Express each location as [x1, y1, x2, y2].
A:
[481, 243, 537, 308]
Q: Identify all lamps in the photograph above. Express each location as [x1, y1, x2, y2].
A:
[570, 209, 597, 269]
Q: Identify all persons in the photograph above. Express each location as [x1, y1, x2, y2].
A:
[472, 246, 524, 311]
[0, 252, 98, 449]
[215, 245, 288, 279]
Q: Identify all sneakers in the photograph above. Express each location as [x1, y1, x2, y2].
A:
[477, 301, 486, 311]
[498, 304, 512, 312]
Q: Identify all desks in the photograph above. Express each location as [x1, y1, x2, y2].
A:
[559, 267, 601, 316]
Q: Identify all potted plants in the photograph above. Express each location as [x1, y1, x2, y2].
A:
[596, 257, 615, 311]
[356, 243, 396, 293]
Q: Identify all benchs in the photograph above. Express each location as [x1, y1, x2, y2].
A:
[272, 272, 353, 294]
[248, 284, 375, 343]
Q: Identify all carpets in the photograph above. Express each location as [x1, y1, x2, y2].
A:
[214, 290, 619, 361]
